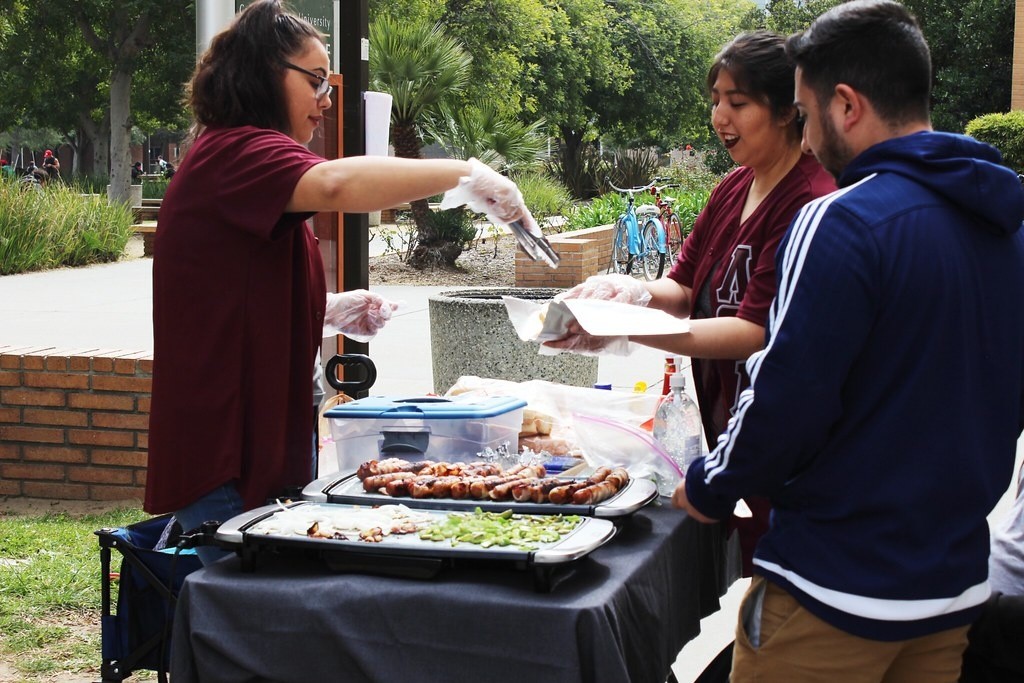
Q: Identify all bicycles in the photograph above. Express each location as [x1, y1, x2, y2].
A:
[605, 175, 670, 283]
[632, 183, 682, 268]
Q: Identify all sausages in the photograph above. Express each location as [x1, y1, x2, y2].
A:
[357, 458, 629, 505]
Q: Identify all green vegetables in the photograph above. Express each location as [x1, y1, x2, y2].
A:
[420, 506, 582, 552]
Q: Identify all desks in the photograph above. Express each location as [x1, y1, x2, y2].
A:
[138, 174, 164, 180]
[171, 488, 741, 683]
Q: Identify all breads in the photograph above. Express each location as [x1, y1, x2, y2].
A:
[516, 409, 584, 458]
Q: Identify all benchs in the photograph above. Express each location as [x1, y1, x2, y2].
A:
[131, 206, 161, 223]
[142, 199, 163, 206]
[130, 224, 156, 256]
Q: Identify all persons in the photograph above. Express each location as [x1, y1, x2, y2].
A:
[131, 161, 143, 185]
[157, 155, 167, 170]
[671, 2, 1023, 683]
[0, 159, 16, 185]
[685, 144, 697, 159]
[42, 150, 61, 182]
[540, 29, 843, 582]
[26, 161, 39, 176]
[143, 0, 536, 548]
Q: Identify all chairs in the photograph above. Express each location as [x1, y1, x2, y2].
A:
[94, 487, 245, 683]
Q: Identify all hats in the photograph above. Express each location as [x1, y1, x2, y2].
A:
[44, 150, 52, 158]
[131, 162, 141, 167]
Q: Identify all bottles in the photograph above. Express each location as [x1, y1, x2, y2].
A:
[653, 374, 703, 496]
[652, 353, 681, 420]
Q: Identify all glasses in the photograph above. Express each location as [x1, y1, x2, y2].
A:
[277, 59, 333, 99]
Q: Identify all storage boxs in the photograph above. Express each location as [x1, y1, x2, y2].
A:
[322, 395, 528, 468]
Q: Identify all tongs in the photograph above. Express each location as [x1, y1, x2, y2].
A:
[506, 219, 560, 269]
